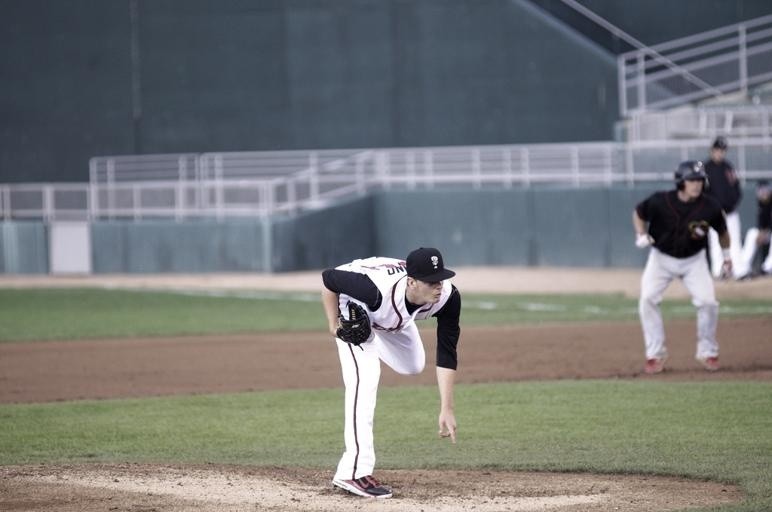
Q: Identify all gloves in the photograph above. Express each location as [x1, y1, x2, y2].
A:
[634, 231, 656, 249]
[716, 260, 733, 281]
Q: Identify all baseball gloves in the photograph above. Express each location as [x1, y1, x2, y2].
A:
[335, 300, 371, 351]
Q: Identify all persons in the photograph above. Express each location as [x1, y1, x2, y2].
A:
[738, 178, 772, 279]
[630, 160, 733, 377]
[319, 243, 462, 501]
[698, 134, 744, 278]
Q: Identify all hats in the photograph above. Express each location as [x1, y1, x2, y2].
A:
[712, 135, 729, 149]
[403, 246, 455, 283]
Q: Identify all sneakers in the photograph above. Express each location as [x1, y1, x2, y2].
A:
[695, 354, 721, 372]
[330, 475, 394, 499]
[674, 160, 711, 193]
[644, 351, 666, 374]
